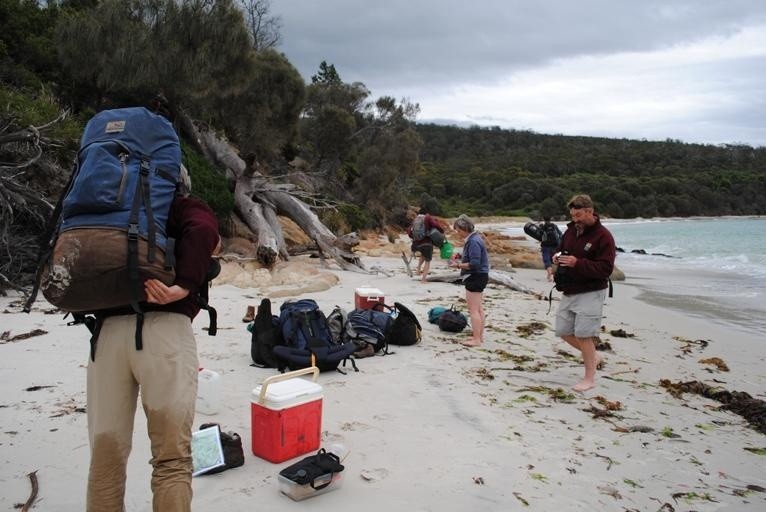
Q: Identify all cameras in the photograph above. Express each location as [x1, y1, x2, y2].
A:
[555, 251, 570, 266]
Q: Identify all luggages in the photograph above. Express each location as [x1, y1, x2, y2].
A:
[412, 215, 426, 241]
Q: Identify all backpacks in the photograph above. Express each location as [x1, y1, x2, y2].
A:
[251, 297, 422, 373]
[543, 224, 559, 245]
[37, 106, 182, 312]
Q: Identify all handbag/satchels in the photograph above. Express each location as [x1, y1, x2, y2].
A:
[200, 422, 245, 478]
[438, 238, 453, 259]
[428, 303, 472, 333]
[428, 227, 444, 248]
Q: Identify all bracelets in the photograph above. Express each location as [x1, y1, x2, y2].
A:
[457, 263, 460, 269]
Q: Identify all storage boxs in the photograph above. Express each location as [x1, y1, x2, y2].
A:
[248, 367, 324, 462]
[355, 287, 385, 310]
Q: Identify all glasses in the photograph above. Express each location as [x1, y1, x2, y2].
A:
[568, 205, 590, 210]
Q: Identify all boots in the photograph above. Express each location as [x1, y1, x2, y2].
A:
[243, 305, 255, 323]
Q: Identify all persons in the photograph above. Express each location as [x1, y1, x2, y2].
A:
[448, 214, 489, 348]
[546, 194, 615, 393]
[539, 215, 562, 283]
[407, 207, 446, 284]
[85, 161, 222, 512]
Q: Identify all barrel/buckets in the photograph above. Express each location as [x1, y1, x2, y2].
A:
[194, 368, 220, 415]
[440, 237, 454, 259]
